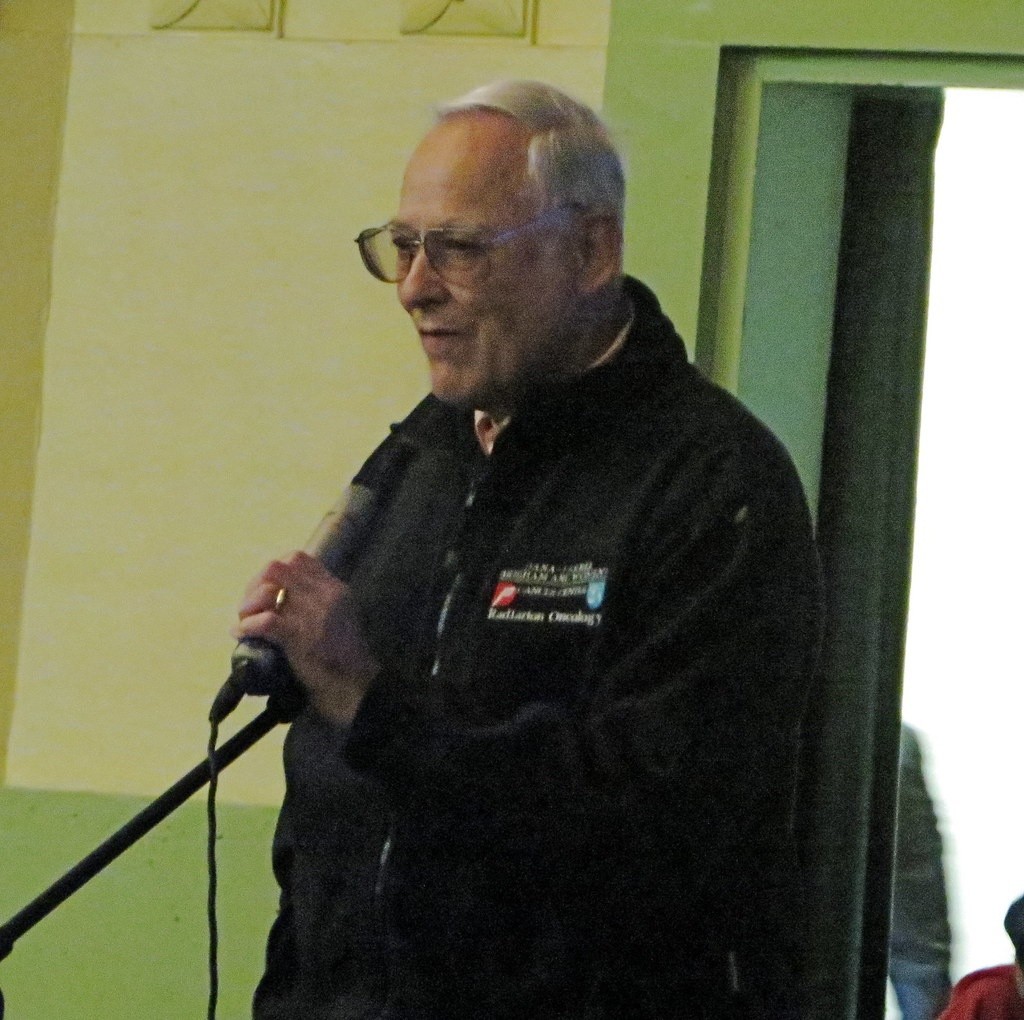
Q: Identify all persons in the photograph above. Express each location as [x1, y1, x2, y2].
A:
[235, 81, 823, 1020]
[888, 725, 1024, 1020]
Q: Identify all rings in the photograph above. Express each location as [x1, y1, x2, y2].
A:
[275, 587, 286, 610]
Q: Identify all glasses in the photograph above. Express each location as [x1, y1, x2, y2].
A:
[355, 204, 581, 291]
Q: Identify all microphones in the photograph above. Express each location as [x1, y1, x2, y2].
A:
[231, 483, 378, 682]
